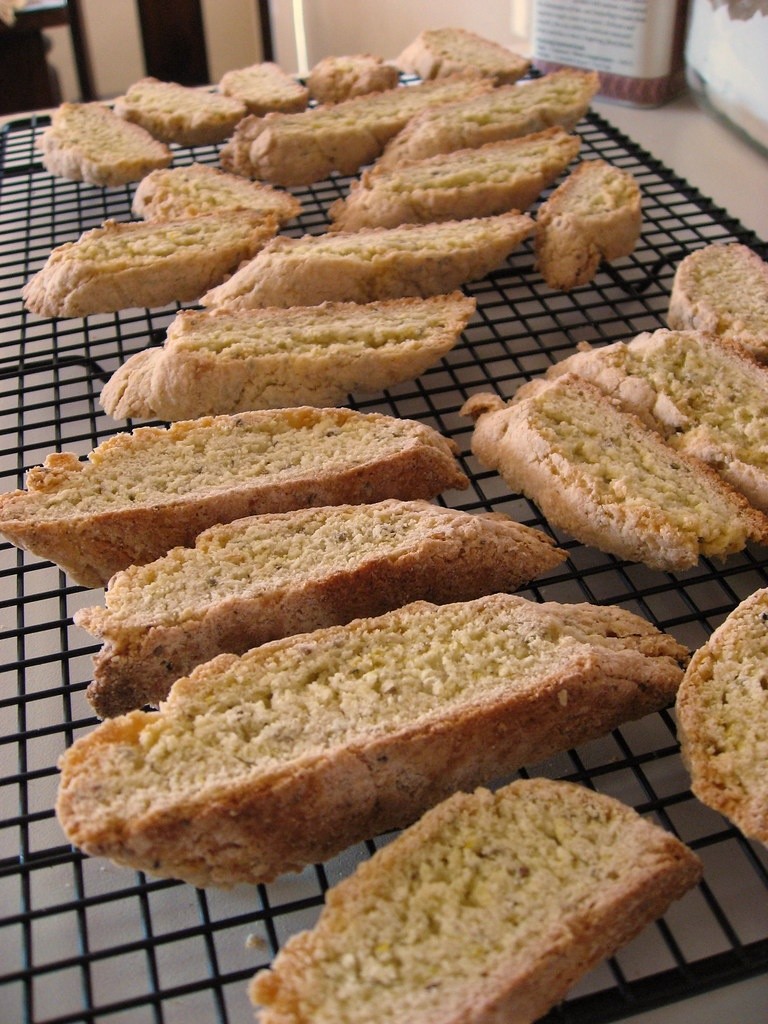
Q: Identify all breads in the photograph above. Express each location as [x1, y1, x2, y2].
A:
[0, 24, 768, 1024]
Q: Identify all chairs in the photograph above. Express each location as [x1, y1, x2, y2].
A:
[65, 0, 276, 102]
